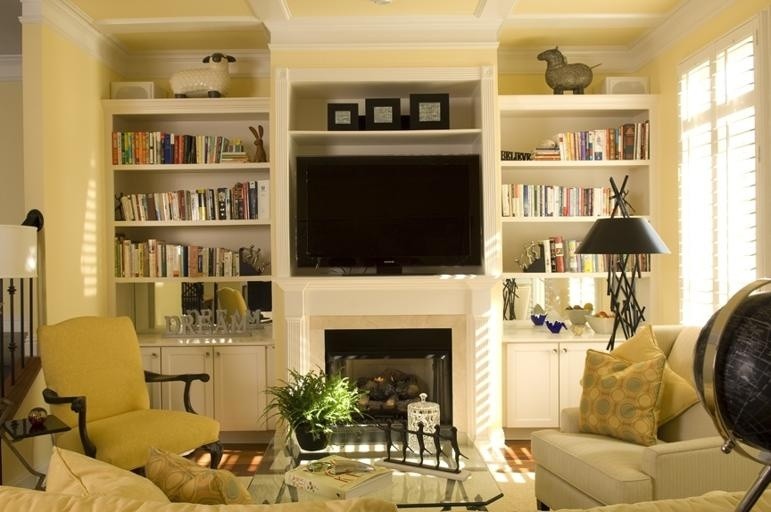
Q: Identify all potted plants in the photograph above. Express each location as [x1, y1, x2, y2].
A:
[260, 366, 366, 452]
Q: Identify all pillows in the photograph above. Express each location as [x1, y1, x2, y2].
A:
[580, 350, 664, 448]
[45, 447, 169, 502]
[143, 446, 255, 507]
[608, 325, 701, 428]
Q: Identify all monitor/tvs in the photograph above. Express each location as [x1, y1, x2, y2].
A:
[296, 154, 485, 276]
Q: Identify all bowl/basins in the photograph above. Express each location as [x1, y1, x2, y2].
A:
[566, 309, 589, 325]
[586, 314, 615, 335]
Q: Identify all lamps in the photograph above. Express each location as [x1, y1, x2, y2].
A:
[574, 175, 671, 353]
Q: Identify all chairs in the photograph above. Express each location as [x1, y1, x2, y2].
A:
[32, 317, 222, 475]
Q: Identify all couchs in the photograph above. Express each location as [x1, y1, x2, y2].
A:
[530, 323, 771, 510]
[0, 485, 398, 512]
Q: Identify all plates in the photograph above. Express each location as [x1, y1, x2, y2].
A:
[216, 288, 248, 322]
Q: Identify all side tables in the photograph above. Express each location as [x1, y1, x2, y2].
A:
[2, 414, 70, 491]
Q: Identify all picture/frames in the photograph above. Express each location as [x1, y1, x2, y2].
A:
[365, 98, 401, 130]
[328, 103, 359, 130]
[409, 92, 450, 129]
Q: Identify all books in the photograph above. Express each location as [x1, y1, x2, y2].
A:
[543, 237, 650, 273]
[120, 178, 271, 222]
[112, 130, 252, 165]
[530, 120, 650, 161]
[501, 183, 623, 216]
[113, 233, 241, 279]
[284, 454, 394, 500]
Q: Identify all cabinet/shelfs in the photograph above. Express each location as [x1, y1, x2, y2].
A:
[505, 335, 625, 441]
[139, 346, 162, 410]
[499, 93, 667, 332]
[107, 95, 273, 342]
[160, 339, 267, 445]
